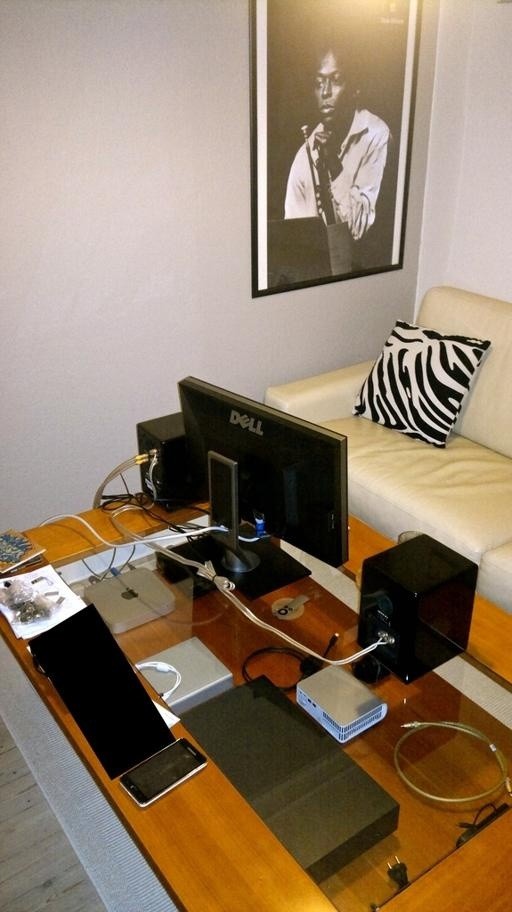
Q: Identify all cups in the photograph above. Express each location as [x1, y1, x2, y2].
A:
[395, 530, 422, 544]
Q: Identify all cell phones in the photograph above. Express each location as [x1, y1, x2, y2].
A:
[119, 736, 209, 808]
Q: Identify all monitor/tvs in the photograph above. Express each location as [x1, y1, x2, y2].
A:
[170, 375, 350, 572]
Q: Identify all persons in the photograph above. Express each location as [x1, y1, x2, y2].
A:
[284, 47, 393, 243]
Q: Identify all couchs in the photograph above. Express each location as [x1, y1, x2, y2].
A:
[260, 281, 511, 616]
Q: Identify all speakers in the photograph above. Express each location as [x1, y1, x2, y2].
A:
[136, 412, 192, 513]
[356, 533, 479, 686]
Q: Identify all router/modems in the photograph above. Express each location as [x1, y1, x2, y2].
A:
[295, 664, 389, 744]
[84, 565, 177, 634]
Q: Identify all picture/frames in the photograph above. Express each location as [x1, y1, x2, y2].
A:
[249, 0, 424, 302]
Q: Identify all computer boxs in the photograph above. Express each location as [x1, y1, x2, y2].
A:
[179, 676, 400, 886]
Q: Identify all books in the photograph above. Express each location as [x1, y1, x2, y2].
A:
[0, 527, 47, 575]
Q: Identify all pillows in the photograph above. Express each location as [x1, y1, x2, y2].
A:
[354, 315, 492, 448]
